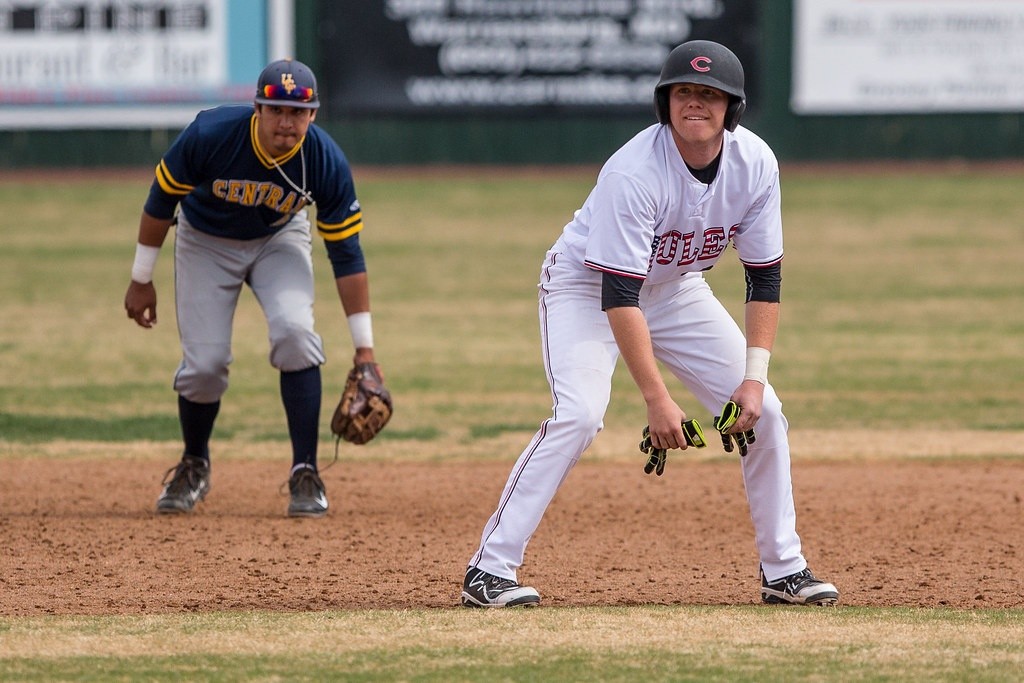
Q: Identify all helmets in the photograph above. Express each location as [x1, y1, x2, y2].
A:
[653, 40, 747, 132]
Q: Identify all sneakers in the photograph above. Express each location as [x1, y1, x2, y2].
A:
[462, 566, 540, 608]
[761, 568, 839, 605]
[280, 470, 328, 518]
[157, 456, 211, 514]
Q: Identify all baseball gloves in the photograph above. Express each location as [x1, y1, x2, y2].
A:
[331, 361, 393, 445]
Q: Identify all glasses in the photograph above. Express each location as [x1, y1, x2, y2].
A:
[256, 84, 318, 103]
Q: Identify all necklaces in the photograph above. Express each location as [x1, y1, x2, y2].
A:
[263, 142, 314, 202]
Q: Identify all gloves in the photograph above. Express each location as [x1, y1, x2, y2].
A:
[640, 419, 708, 476]
[713, 401, 756, 457]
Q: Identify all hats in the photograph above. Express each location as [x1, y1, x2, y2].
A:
[255, 57, 320, 108]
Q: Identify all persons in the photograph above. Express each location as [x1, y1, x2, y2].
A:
[461, 41, 839, 609]
[124, 59, 392, 517]
